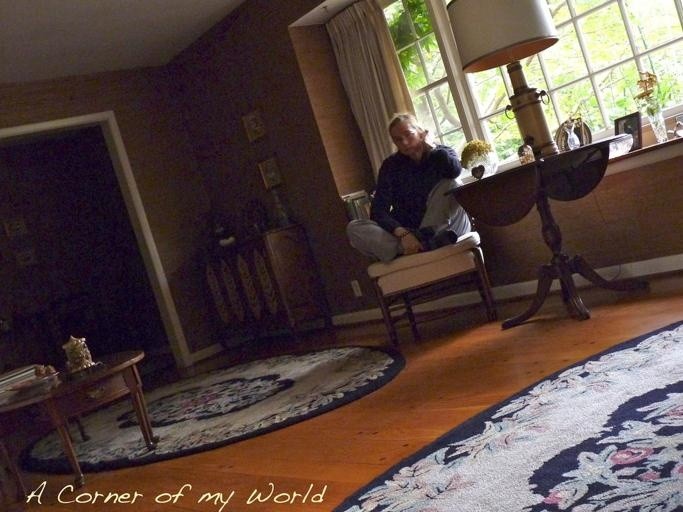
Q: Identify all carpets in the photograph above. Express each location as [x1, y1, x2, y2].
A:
[331, 321, 683, 512]
[18, 344, 406, 473]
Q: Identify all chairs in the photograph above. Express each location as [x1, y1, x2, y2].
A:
[366, 187, 497, 347]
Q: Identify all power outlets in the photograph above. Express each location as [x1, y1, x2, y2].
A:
[351, 280, 362, 297]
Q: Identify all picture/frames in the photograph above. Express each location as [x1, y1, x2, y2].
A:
[257, 158, 285, 191]
[614, 113, 643, 150]
[241, 108, 268, 143]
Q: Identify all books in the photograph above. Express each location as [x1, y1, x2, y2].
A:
[0, 364, 37, 395]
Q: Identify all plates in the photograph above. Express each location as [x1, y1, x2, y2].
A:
[554, 119, 592, 154]
[5, 371, 60, 392]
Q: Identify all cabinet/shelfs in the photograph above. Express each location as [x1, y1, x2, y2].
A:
[176, 223, 334, 356]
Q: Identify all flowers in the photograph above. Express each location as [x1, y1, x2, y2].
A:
[632, 71, 673, 115]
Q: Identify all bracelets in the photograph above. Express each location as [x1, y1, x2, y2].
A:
[398, 230, 411, 241]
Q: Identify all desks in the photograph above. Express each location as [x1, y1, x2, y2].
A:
[443, 134, 649, 329]
[0, 349, 160, 502]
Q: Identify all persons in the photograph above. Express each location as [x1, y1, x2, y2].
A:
[346, 113, 472, 265]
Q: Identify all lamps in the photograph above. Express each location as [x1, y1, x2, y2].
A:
[447, 0, 575, 159]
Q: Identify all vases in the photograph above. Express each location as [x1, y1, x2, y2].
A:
[648, 112, 668, 143]
